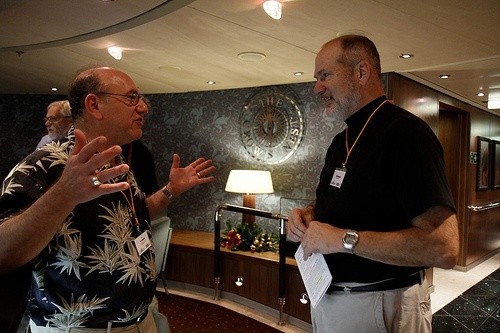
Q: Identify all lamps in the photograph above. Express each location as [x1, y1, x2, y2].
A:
[263, 0, 281, 19]
[225, 170, 275, 227]
[487, 92, 500, 109]
[107, 47, 122, 60]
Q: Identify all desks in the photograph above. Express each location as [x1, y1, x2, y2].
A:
[168, 229, 312, 333]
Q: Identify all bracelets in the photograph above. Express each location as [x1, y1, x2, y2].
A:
[162, 186, 176, 202]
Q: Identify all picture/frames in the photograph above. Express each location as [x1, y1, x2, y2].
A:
[491, 140, 500, 190]
[476, 136, 491, 192]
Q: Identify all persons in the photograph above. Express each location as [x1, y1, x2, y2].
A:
[285, 34, 459, 333]
[36, 100, 73, 149]
[0, 67, 215, 333]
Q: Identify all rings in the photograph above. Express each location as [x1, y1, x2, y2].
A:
[197, 173, 201, 177]
[92, 176, 101, 186]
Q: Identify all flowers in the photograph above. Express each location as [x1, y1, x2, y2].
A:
[221, 218, 279, 253]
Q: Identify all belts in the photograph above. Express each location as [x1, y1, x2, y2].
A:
[83, 306, 150, 329]
[326, 270, 426, 296]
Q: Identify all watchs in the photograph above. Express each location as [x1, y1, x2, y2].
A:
[342, 230, 359, 254]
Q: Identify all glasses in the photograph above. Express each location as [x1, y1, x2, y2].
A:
[44, 114, 74, 124]
[93, 87, 151, 111]
